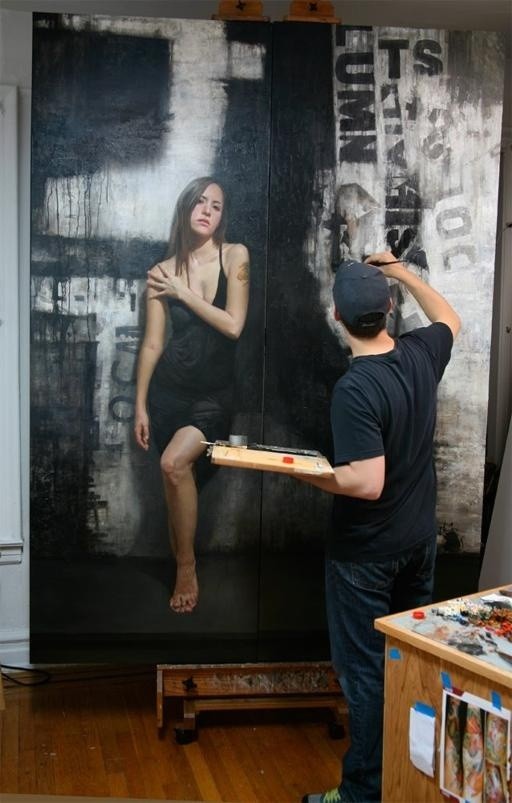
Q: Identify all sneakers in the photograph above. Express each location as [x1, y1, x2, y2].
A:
[303, 787, 343, 803]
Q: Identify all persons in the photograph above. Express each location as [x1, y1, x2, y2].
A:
[130, 173, 253, 614]
[283, 250, 462, 803]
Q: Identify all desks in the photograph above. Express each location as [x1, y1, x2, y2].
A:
[371, 583, 509, 803]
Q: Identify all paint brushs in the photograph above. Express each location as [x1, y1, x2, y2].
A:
[200, 440, 318, 457]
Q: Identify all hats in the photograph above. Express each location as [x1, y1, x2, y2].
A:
[333, 260, 391, 329]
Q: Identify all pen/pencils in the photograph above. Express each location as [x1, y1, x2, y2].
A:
[370, 260, 408, 266]
